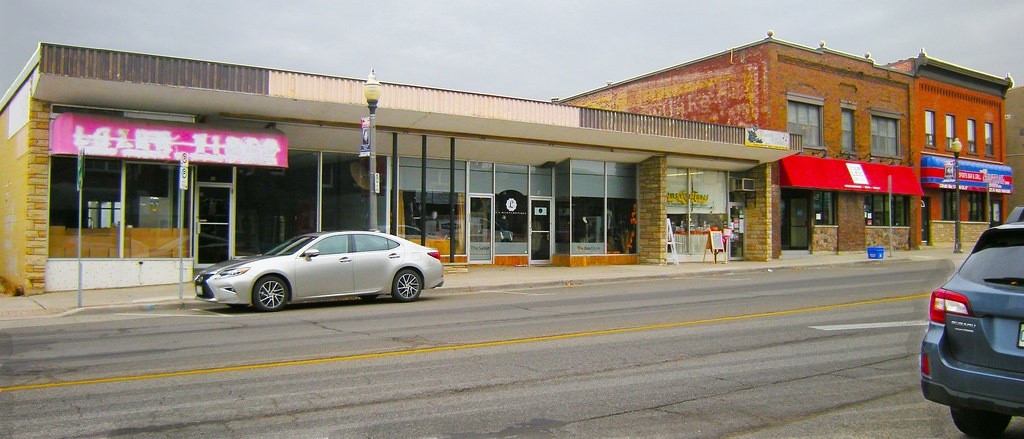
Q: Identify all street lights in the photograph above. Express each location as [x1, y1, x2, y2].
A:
[364, 69, 383, 234]
[951, 137, 964, 254]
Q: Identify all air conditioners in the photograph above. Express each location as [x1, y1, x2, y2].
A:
[735, 178, 755, 192]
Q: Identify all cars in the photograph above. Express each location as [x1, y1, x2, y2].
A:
[377, 224, 441, 239]
[433, 217, 514, 243]
[54, 233, 150, 258]
[149, 233, 229, 263]
[919, 220, 1024, 438]
[194, 230, 445, 312]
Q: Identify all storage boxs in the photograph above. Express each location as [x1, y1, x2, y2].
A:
[867, 248, 884, 259]
